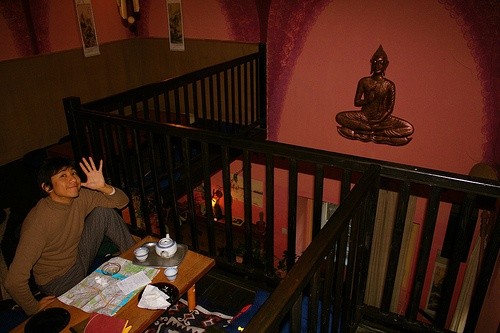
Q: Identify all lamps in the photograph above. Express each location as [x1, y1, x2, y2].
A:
[211, 190, 222, 208]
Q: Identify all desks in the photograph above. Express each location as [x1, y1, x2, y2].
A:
[9, 235, 216, 333]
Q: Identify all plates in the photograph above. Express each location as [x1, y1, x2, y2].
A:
[24, 307, 71, 333]
[138, 282, 179, 310]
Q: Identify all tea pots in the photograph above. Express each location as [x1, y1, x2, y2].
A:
[155, 234, 177, 258]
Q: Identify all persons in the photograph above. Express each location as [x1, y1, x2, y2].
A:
[4, 155, 137, 317]
[334, 43, 414, 146]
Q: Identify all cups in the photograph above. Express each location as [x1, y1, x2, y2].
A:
[164, 268, 178, 282]
[133, 247, 149, 263]
